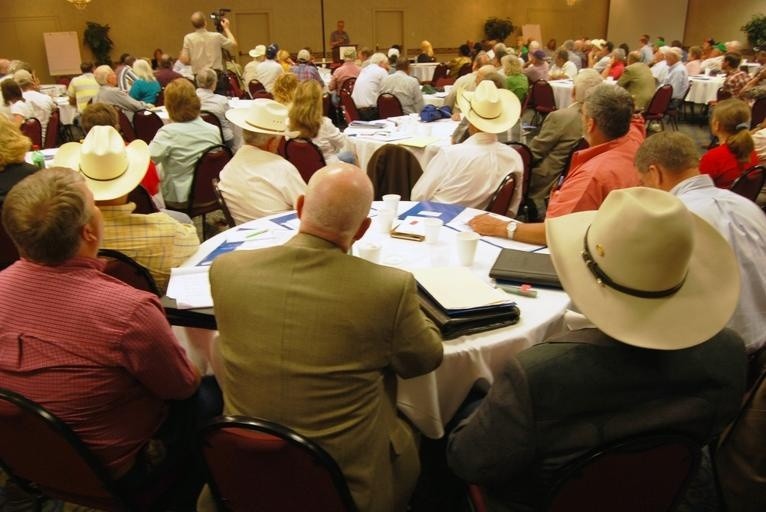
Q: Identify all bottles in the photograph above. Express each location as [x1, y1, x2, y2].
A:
[31, 145, 46, 168]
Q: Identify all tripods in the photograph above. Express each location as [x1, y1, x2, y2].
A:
[215, 22, 254, 100]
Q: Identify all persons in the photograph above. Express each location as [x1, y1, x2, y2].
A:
[1, 11, 765, 511]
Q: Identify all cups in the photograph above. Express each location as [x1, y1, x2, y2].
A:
[382, 193, 401, 221]
[357, 241, 381, 264]
[454, 231, 479, 265]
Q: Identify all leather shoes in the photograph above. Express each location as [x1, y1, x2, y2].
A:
[702, 143, 720, 149]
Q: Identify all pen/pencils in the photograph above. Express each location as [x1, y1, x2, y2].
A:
[489, 284, 537, 298]
[245, 228, 269, 238]
[464, 212, 490, 225]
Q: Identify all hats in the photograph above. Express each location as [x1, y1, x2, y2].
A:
[223, 98, 300, 138]
[388, 48, 400, 60]
[456, 80, 521, 134]
[343, 48, 358, 61]
[659, 46, 671, 55]
[249, 45, 267, 58]
[592, 38, 607, 50]
[543, 186, 741, 349]
[51, 125, 151, 201]
[296, 49, 310, 62]
[531, 51, 547, 61]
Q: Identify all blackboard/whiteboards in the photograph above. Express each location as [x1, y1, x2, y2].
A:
[522, 24, 543, 47]
[43, 31, 83, 76]
